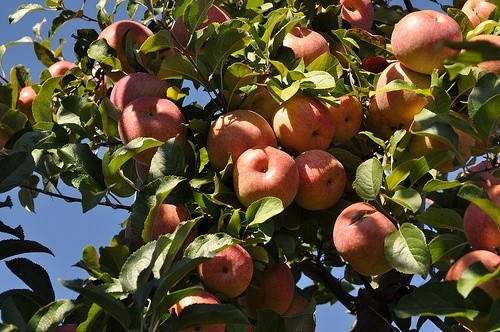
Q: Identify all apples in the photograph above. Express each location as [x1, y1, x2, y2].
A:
[0, 0, 500, 332]
